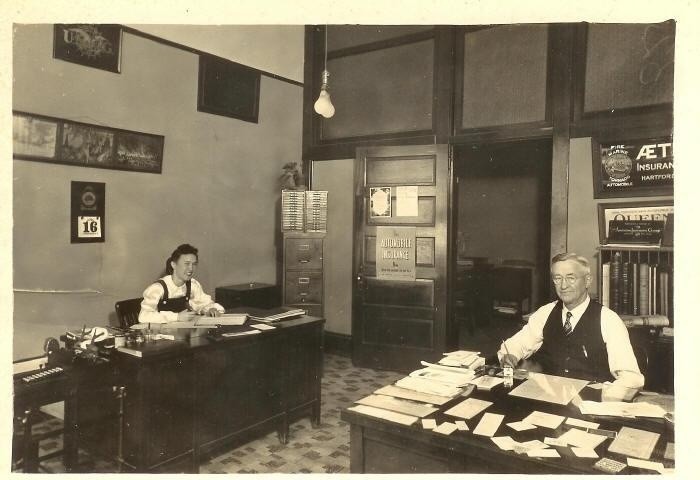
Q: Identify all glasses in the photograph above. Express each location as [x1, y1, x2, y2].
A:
[552, 275, 586, 285]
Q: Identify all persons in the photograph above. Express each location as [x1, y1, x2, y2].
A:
[496, 252, 646, 391]
[138, 244, 226, 324]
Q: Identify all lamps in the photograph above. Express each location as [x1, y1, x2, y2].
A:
[314, 24, 336, 119]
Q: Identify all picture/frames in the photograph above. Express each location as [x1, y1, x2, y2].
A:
[53, 24, 122, 73]
[12, 108, 165, 174]
[598, 200, 673, 245]
[197, 52, 259, 124]
[592, 127, 673, 199]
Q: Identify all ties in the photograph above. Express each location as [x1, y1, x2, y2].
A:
[564, 312, 572, 336]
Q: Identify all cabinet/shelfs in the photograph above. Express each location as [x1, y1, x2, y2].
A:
[283, 231, 326, 356]
[217, 283, 279, 309]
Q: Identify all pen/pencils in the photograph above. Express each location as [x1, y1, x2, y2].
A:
[502, 341, 513, 363]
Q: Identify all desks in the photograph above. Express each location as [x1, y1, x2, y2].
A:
[61, 307, 328, 474]
[342, 361, 674, 474]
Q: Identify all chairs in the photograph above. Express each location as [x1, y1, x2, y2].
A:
[115, 299, 143, 328]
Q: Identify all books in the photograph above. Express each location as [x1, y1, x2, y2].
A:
[195, 313, 253, 326]
[246, 304, 306, 322]
[600, 252, 675, 329]
